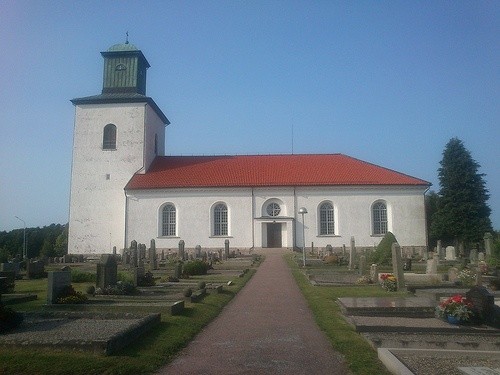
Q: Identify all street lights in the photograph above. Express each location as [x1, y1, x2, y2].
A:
[298, 208, 309, 267]
[15, 216, 27, 257]
